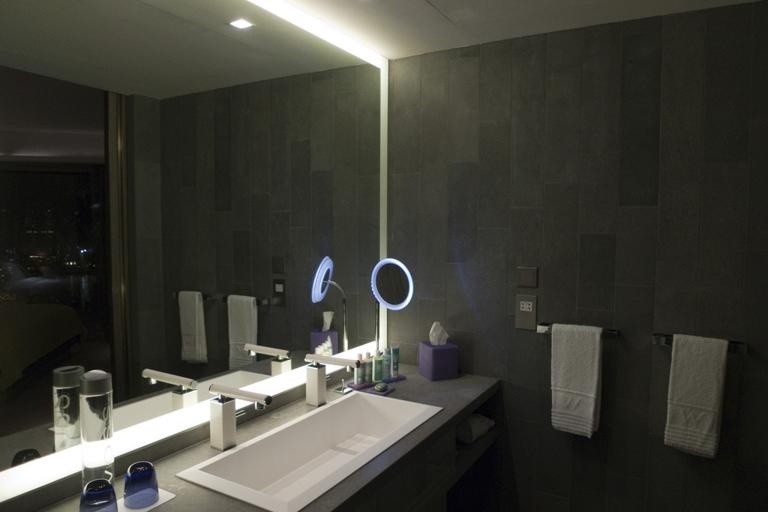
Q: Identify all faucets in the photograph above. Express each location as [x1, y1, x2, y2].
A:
[141, 368, 198, 409]
[209, 384, 272, 450]
[303, 354, 360, 406]
[244, 343, 292, 376]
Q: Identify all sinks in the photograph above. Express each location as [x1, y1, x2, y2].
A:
[175, 390, 443, 512]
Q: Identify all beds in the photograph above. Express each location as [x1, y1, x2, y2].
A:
[0, 262, 89, 312]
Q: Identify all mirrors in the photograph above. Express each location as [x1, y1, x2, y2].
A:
[0, 1, 389, 512]
[371, 259, 414, 350]
[310, 254, 349, 351]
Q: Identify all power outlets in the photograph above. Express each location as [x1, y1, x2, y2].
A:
[514, 294, 538, 332]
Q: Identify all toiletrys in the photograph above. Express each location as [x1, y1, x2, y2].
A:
[314, 336, 333, 358]
[353, 346, 401, 386]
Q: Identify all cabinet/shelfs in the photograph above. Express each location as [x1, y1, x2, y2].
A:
[337, 362, 504, 512]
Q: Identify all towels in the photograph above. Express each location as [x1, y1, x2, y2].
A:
[177, 291, 208, 363]
[227, 295, 257, 370]
[665, 333, 729, 462]
[550, 323, 603, 439]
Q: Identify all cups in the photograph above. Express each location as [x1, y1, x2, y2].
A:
[13, 447, 40, 468]
[123, 461, 159, 509]
[79, 478, 117, 512]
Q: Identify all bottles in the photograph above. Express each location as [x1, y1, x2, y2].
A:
[78, 368, 118, 492]
[51, 364, 84, 451]
